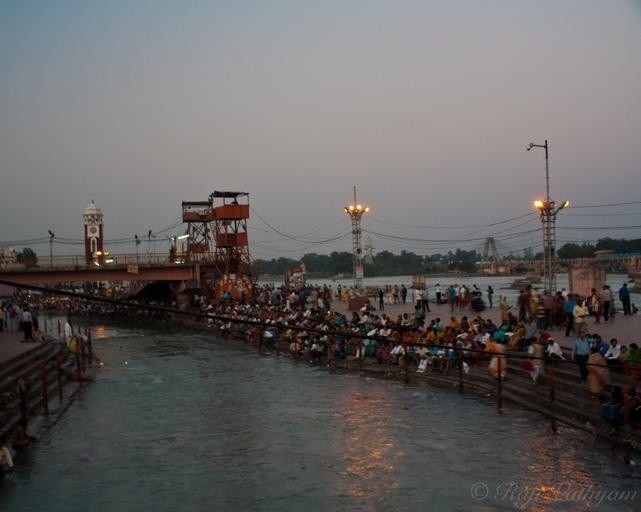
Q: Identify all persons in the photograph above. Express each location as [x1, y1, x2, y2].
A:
[0, 243, 641, 475]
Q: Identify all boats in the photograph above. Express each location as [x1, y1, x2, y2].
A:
[615, 273, 641, 293]
[499, 272, 544, 289]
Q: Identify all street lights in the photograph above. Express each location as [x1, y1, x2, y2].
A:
[527, 139, 569, 296]
[48, 229, 54, 266]
[344, 185, 369, 289]
[134, 230, 153, 263]
